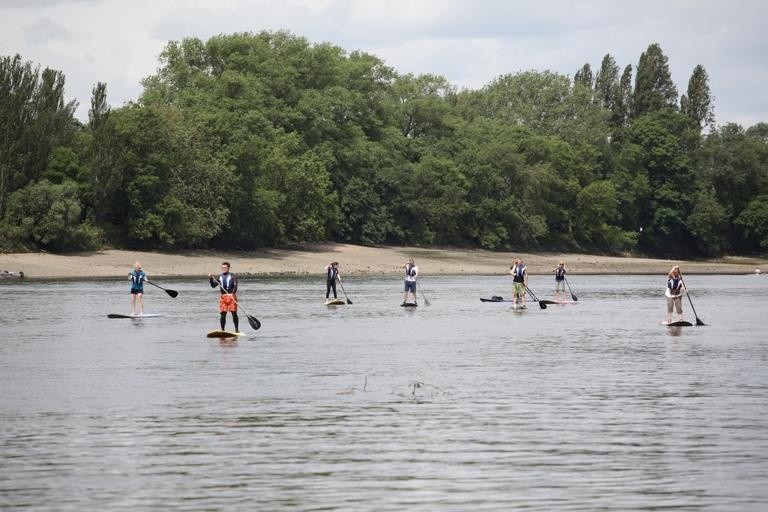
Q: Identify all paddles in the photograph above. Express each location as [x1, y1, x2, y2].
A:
[413, 275, 431, 306]
[522, 283, 547, 309]
[564, 277, 577, 301]
[336, 273, 353, 304]
[212, 275, 261, 330]
[130, 274, 178, 298]
[679, 270, 704, 325]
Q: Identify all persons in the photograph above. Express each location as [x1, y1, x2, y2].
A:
[401, 258, 418, 304]
[323, 261, 342, 304]
[552, 260, 569, 301]
[509, 256, 522, 305]
[127, 261, 147, 316]
[663, 265, 689, 324]
[207, 261, 240, 333]
[510, 257, 528, 309]
